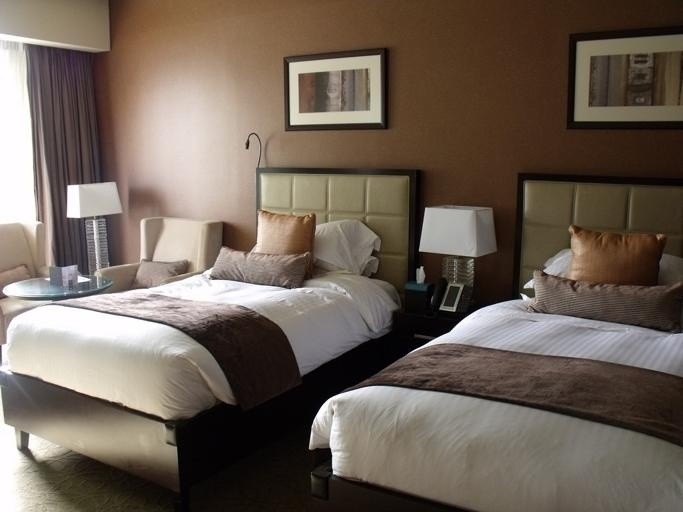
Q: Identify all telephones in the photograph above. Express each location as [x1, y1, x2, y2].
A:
[430, 277, 472, 317]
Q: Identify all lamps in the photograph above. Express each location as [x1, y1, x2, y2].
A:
[244, 133, 263, 169]
[417, 201, 502, 286]
[65, 181, 124, 274]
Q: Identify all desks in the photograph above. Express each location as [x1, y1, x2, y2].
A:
[1, 269, 115, 301]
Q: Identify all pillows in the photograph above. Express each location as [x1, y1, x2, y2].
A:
[210, 244, 315, 290]
[128, 257, 191, 289]
[565, 224, 667, 284]
[525, 270, 681, 333]
[248, 218, 381, 279]
[254, 207, 316, 256]
[0, 262, 32, 299]
[522, 245, 682, 291]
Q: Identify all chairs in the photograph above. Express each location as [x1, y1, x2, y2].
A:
[95, 214, 224, 294]
[0, 219, 94, 344]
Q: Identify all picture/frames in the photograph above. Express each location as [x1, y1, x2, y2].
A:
[281, 46, 390, 132]
[566, 23, 683, 131]
[438, 281, 464, 312]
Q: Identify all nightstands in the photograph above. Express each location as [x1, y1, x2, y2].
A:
[395, 306, 469, 340]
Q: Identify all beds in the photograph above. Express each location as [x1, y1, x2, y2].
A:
[0, 165, 428, 509]
[300, 173, 680, 511]
